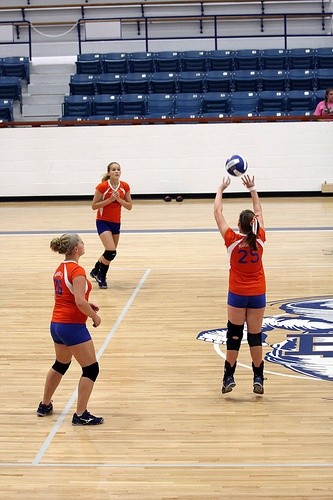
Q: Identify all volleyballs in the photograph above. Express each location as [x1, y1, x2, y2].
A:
[224, 153, 249, 177]
[176, 196, 182, 202]
[163, 196, 171, 202]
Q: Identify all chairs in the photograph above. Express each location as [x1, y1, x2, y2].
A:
[58, 48, 333, 127]
[0, 56, 30, 128]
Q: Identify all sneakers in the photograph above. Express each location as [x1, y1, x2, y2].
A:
[90, 269, 99, 280]
[36, 400, 53, 416]
[221, 376, 235, 393]
[96, 272, 107, 288]
[253, 376, 267, 394]
[72, 409, 103, 426]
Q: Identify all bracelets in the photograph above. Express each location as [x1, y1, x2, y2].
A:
[248, 185, 256, 192]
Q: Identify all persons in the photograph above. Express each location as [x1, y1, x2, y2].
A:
[313, 89, 333, 116]
[213, 175, 265, 395]
[38, 232, 105, 425]
[91, 162, 132, 289]
